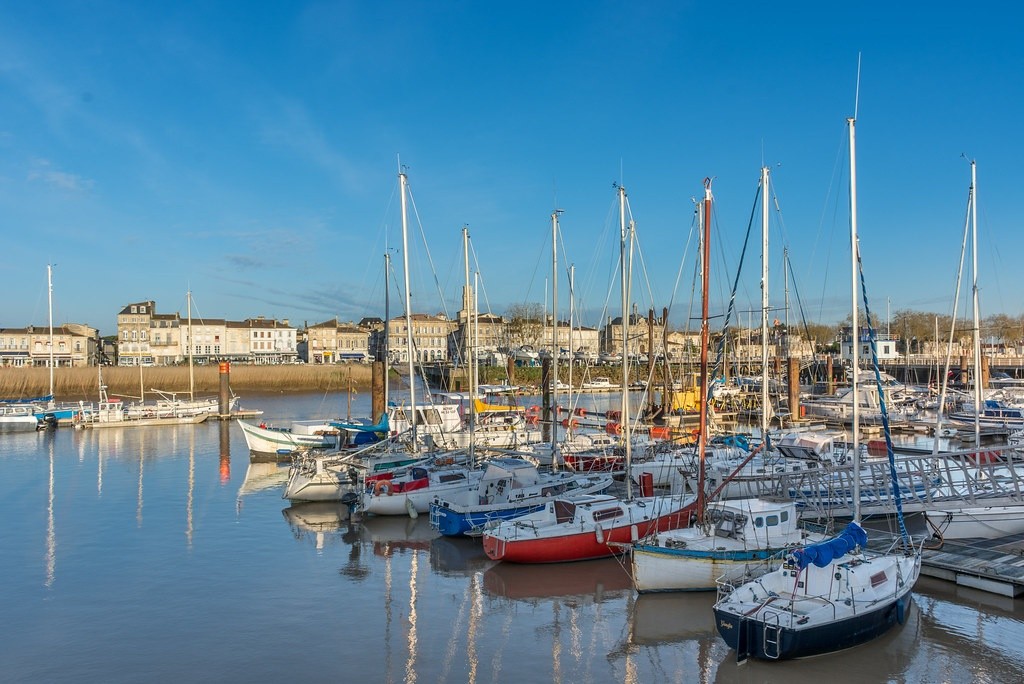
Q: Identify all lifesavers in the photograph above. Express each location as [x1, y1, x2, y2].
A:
[374, 480, 394, 497]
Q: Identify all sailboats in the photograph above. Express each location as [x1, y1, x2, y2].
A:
[0, 261, 265, 430]
[234, 43, 1024, 669]
